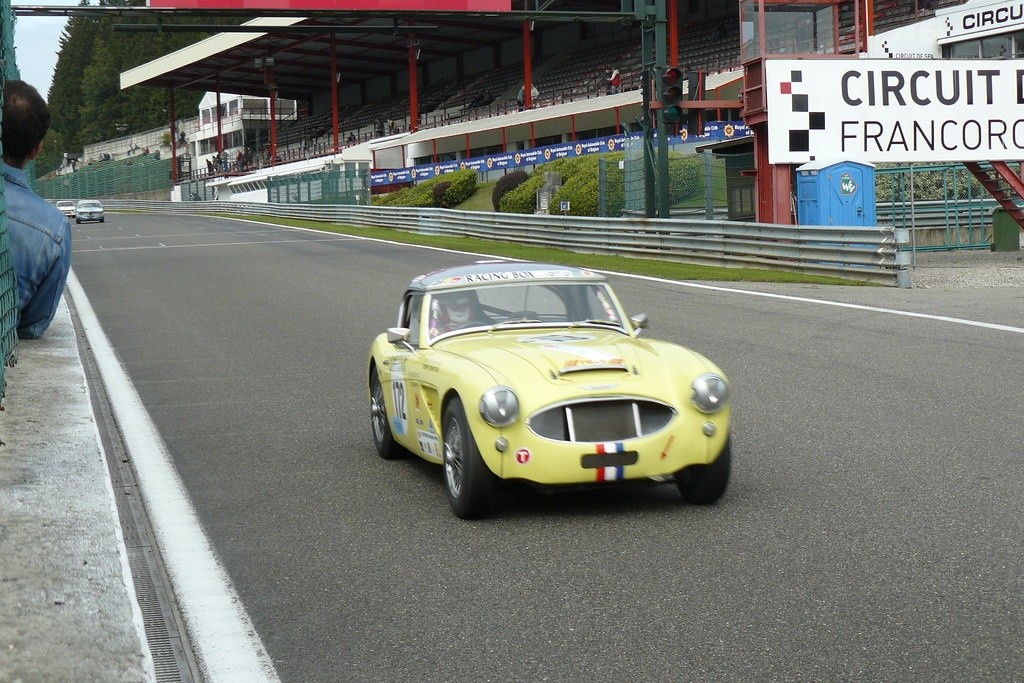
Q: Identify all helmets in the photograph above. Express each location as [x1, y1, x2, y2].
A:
[446, 296, 471, 323]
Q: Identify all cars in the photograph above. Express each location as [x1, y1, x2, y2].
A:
[56, 200, 76, 218]
[367, 263, 732, 521]
[75, 199, 105, 224]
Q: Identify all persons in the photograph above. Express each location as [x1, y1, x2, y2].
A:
[408, 116, 411, 131]
[263, 141, 282, 168]
[682, 66, 689, 80]
[517, 85, 525, 112]
[47, 130, 186, 180]
[428, 293, 491, 339]
[206, 147, 253, 179]
[346, 132, 356, 146]
[531, 84, 539, 109]
[0, 80, 72, 339]
[373, 119, 399, 138]
[602, 65, 620, 96]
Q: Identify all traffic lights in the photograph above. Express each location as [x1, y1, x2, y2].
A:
[660, 67, 685, 120]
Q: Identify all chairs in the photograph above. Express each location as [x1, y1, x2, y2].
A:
[242, 0, 970, 169]
